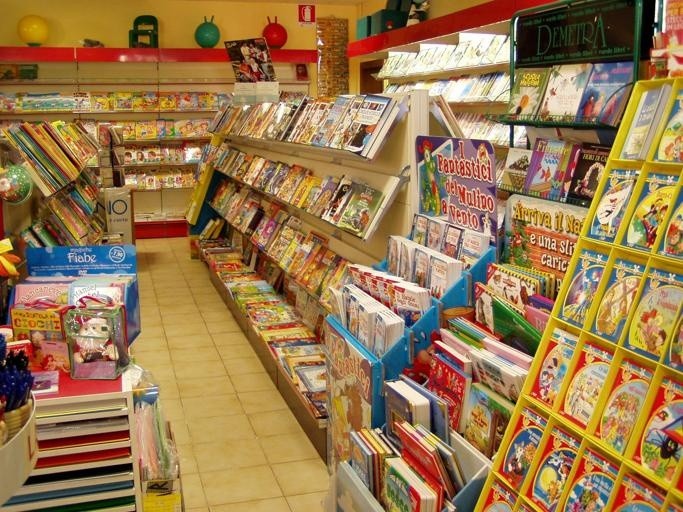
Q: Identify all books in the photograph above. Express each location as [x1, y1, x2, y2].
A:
[2, 91, 229, 108]
[337, 32, 682, 511]
[123, 144, 203, 161]
[125, 168, 194, 187]
[0, 120, 179, 512]
[211, 142, 385, 238]
[84, 119, 212, 140]
[199, 218, 332, 419]
[211, 178, 352, 313]
[209, 95, 407, 160]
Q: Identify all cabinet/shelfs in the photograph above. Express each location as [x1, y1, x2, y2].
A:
[0, 36, 316, 240]
[1, 116, 138, 511]
[193, 0, 683, 512]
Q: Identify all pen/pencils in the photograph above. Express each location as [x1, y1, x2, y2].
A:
[0, 333, 34, 421]
